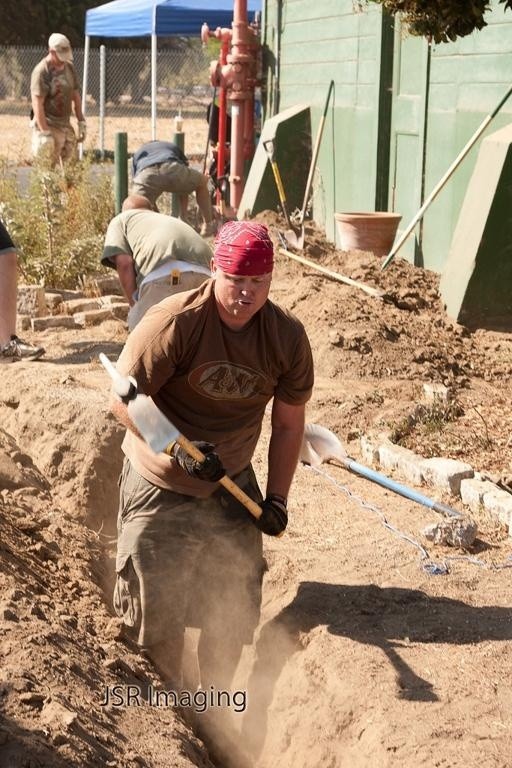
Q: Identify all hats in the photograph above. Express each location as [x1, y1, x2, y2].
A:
[48, 32, 74, 62]
[214, 221, 273, 276]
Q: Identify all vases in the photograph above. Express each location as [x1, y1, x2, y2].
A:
[334, 211, 402, 259]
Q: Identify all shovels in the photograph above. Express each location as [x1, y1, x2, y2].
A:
[297, 80, 334, 250]
[263, 139, 302, 249]
[299, 424, 461, 516]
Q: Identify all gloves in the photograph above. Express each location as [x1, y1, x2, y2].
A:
[77, 121, 87, 143]
[174, 441, 226, 483]
[252, 497, 288, 536]
[39, 131, 55, 157]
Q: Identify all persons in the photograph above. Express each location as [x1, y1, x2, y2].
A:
[101, 194, 213, 332]
[107, 220, 312, 710]
[30, 32, 89, 208]
[0, 221, 46, 362]
[128, 140, 216, 237]
[207, 92, 232, 175]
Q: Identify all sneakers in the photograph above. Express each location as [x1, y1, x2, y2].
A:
[200, 223, 217, 237]
[0, 335, 46, 362]
